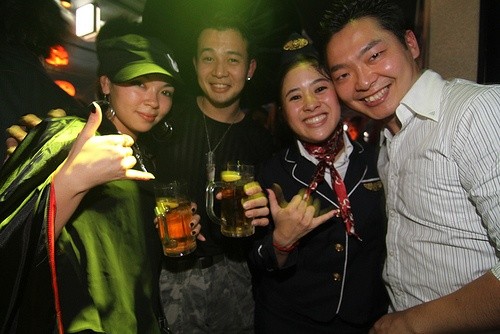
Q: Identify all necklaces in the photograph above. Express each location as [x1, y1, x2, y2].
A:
[201, 102, 238, 180]
[119, 131, 152, 183]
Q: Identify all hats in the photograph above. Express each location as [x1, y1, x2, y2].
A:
[98, 34, 181, 83]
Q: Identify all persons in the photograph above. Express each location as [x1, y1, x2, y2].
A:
[1, 0, 500, 334]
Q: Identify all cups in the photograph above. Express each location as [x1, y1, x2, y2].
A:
[153, 178, 197, 257]
[205, 159, 258, 238]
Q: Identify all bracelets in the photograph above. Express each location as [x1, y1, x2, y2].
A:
[273, 238, 297, 254]
